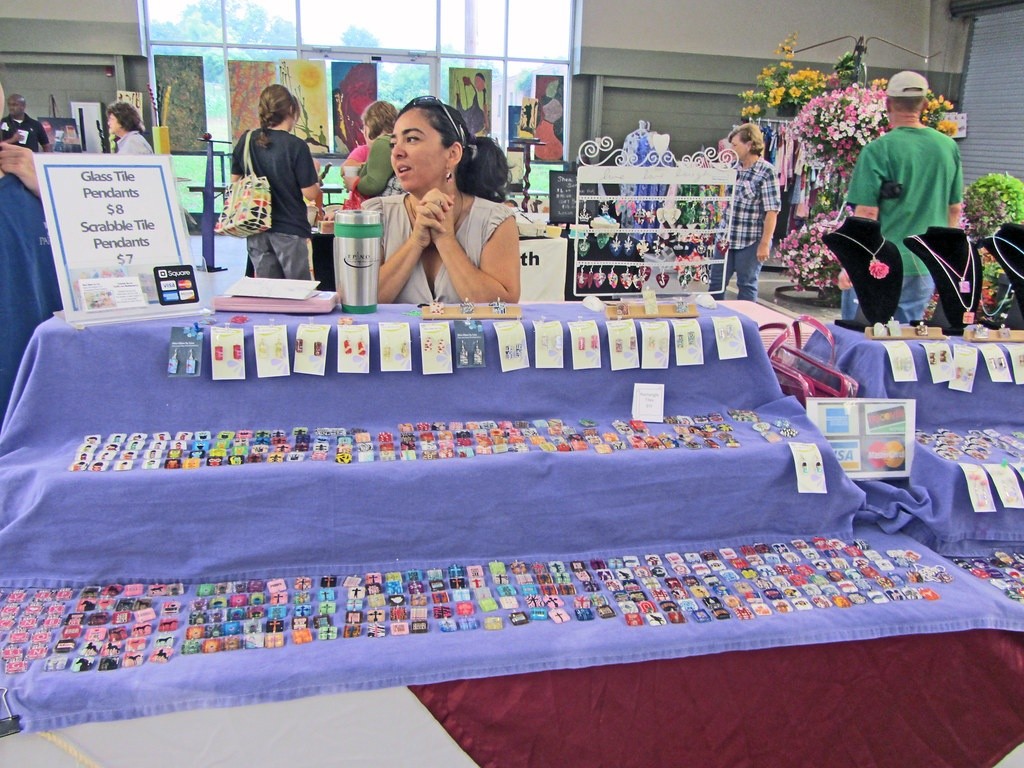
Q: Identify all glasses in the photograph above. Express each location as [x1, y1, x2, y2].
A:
[358, 123, 366, 133]
[399, 95, 461, 140]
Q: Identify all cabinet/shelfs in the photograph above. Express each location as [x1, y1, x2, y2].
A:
[0, 297, 1023, 768]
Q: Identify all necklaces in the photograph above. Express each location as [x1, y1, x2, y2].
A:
[408, 190, 464, 226]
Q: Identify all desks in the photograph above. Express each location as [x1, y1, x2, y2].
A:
[187, 184, 342, 206]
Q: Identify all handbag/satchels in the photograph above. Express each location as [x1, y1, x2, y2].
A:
[761, 315, 859, 409]
[214, 130, 272, 239]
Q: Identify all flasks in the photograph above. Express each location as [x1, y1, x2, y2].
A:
[332, 209, 384, 315]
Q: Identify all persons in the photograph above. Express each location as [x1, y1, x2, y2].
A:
[707, 123, 781, 302]
[104, 100, 154, 155]
[0, 85, 54, 430]
[361, 94, 521, 307]
[231, 84, 320, 281]
[838, 71, 965, 325]
[339, 101, 407, 210]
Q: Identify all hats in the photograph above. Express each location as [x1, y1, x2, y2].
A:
[885, 70, 929, 97]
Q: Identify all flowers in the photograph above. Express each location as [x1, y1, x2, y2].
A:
[961, 172, 1024, 245]
[739, 30, 959, 298]
